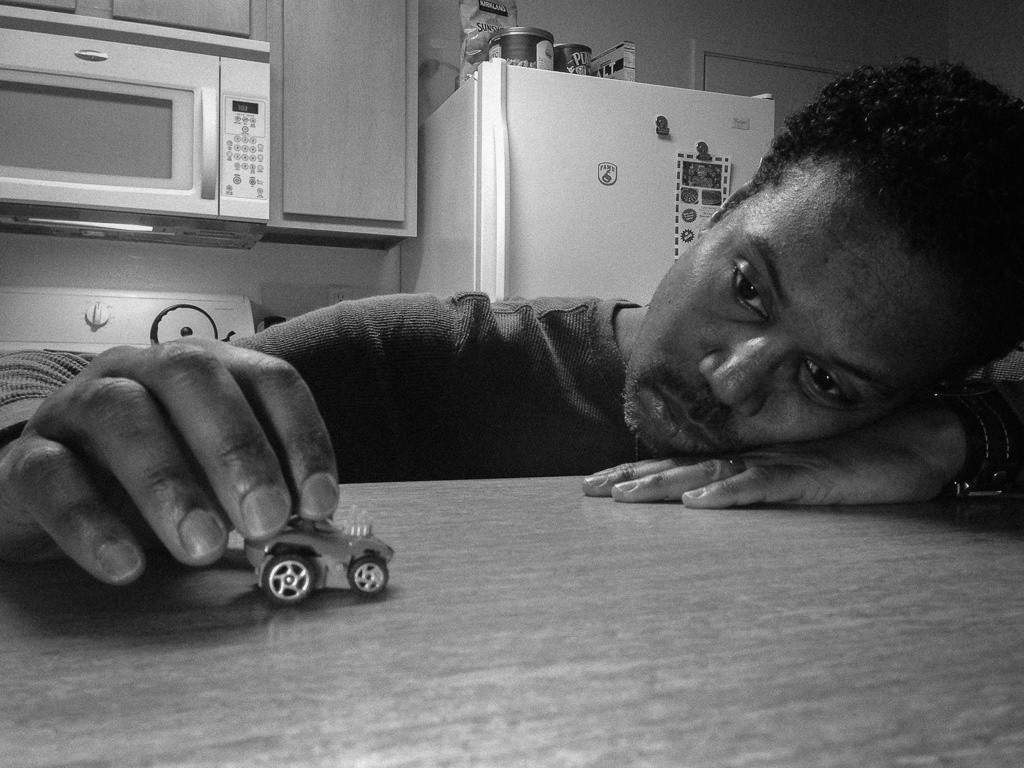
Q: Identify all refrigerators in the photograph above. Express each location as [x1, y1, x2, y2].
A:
[396, 57, 775, 325]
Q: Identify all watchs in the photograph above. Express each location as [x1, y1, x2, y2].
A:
[928, 383, 1014, 502]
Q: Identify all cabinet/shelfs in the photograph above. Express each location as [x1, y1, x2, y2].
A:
[254, 0, 419, 249]
[0, 1, 253, 39]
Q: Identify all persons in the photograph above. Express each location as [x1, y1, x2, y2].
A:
[0, 53, 1024, 595]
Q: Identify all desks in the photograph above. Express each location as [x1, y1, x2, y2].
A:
[1, 473, 1024, 766]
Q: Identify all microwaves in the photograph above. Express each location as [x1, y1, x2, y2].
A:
[0, 4, 273, 253]
